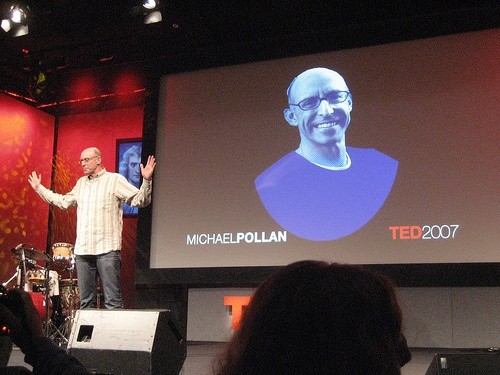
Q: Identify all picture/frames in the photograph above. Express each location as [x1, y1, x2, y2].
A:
[115, 138, 142, 218]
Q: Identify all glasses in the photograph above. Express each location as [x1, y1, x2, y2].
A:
[286, 91, 350, 112]
[78, 155, 99, 164]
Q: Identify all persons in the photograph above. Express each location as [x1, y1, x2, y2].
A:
[217, 260, 411, 375]
[28, 147, 156, 309]
[0, 284, 90, 375]
[255, 67, 398, 241]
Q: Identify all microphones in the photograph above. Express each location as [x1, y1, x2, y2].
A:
[14, 243, 24, 251]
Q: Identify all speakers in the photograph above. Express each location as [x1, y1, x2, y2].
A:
[424, 351, 500, 375]
[67, 308, 186, 375]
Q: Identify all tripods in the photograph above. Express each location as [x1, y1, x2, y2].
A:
[19, 249, 68, 343]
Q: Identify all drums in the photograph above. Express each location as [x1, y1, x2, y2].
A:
[52, 242, 74, 261]
[27, 271, 46, 284]
[59, 278, 80, 316]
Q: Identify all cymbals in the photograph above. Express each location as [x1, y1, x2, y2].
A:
[11, 247, 50, 262]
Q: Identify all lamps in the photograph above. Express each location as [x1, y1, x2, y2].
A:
[1, 1, 30, 36]
[129, 0, 162, 24]
